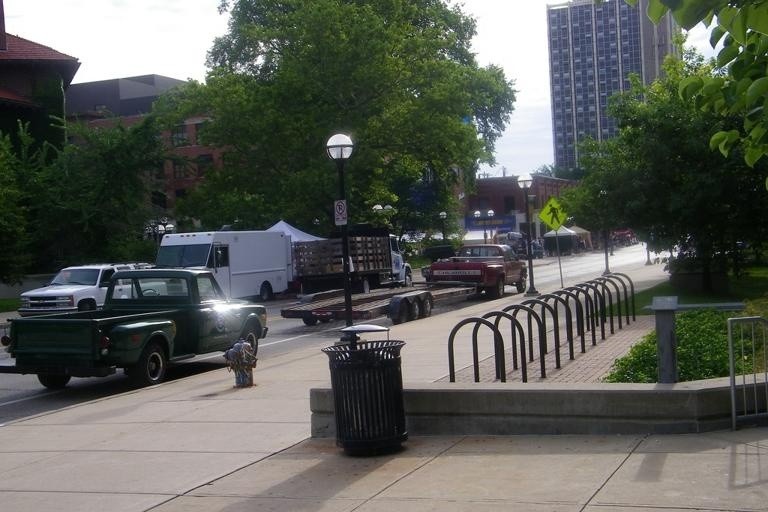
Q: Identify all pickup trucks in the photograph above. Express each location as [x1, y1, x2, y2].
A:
[428, 243, 528, 296]
[6, 267, 268, 387]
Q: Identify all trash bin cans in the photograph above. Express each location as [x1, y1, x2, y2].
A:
[322, 340, 408, 456]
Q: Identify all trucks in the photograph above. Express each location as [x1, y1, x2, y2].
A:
[293, 232, 413, 294]
[154, 230, 294, 301]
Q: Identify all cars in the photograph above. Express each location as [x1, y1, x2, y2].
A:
[17, 262, 168, 317]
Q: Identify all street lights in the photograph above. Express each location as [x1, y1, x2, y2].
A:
[474, 209, 494, 243]
[438, 211, 447, 243]
[517, 173, 541, 295]
[325, 132, 368, 347]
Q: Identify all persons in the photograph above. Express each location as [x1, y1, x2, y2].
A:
[164, 278, 188, 297]
[529, 235, 546, 259]
